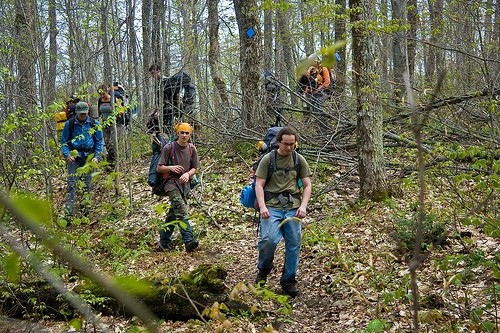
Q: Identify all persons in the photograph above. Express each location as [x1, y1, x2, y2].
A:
[255, 126, 313, 299]
[62, 98, 80, 110]
[60, 102, 102, 226]
[157, 123, 199, 253]
[149, 64, 169, 118]
[296, 56, 330, 139]
[97, 85, 124, 171]
[109, 82, 124, 99]
[264, 68, 281, 127]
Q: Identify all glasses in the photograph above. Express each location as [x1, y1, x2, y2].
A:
[282, 143, 293, 146]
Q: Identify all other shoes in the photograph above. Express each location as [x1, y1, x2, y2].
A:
[285, 284, 296, 295]
[186, 240, 197, 253]
[256, 270, 268, 286]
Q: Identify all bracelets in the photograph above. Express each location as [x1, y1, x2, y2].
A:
[187, 172, 190, 176]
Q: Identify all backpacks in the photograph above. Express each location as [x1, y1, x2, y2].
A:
[148, 143, 193, 196]
[319, 67, 334, 94]
[253, 127, 299, 189]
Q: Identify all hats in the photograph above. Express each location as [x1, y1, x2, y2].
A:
[175, 122, 193, 132]
[75, 102, 88, 114]
